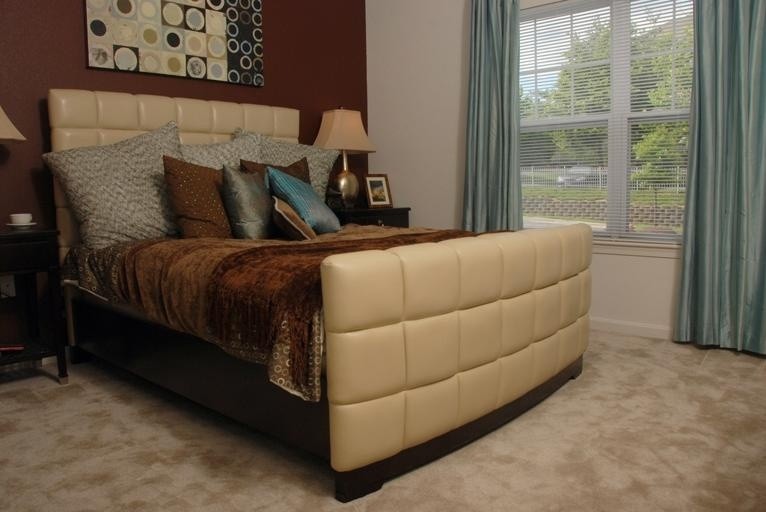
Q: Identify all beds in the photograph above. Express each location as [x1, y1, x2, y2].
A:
[47, 88, 592, 504]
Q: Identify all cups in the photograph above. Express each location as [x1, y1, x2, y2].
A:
[13, 226, 30, 230]
[8, 213, 33, 225]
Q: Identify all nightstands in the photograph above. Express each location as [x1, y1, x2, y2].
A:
[0, 228, 69, 386]
[335, 207, 412, 227]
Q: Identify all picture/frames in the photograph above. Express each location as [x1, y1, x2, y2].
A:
[361, 173, 393, 209]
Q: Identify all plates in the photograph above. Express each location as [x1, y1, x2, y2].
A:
[8, 229, 33, 233]
[3, 223, 38, 226]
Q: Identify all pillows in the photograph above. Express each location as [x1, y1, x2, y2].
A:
[180, 135, 261, 170]
[162, 154, 234, 238]
[267, 167, 341, 233]
[222, 164, 273, 239]
[41, 120, 186, 249]
[233, 126, 340, 201]
[240, 157, 311, 186]
[272, 196, 317, 241]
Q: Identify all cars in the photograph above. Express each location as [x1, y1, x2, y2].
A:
[556, 163, 607, 188]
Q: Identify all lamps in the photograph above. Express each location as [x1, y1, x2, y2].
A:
[0, 106, 29, 145]
[312, 106, 376, 208]
